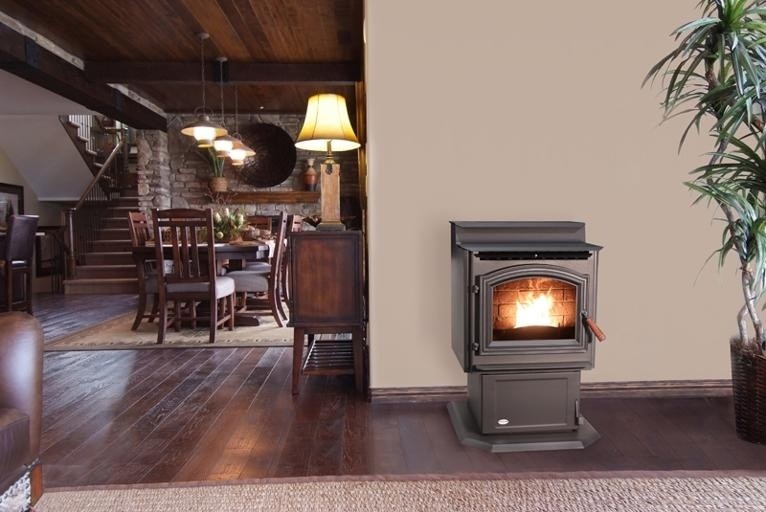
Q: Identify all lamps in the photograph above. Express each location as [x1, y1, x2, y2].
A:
[179, 28, 257, 166]
[293, 90, 360, 231]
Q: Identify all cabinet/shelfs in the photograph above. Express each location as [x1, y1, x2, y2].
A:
[284, 230, 366, 397]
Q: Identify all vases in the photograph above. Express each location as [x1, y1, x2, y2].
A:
[303, 158, 318, 192]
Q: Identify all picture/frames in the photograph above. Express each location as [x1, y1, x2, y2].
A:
[0, 182, 25, 233]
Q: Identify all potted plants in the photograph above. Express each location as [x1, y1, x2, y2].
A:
[195, 147, 228, 192]
[638, 1, 765, 445]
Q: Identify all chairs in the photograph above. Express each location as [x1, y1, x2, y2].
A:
[125, 208, 303, 345]
[0, 214, 40, 317]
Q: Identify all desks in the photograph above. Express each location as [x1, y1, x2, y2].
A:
[1, 230, 46, 313]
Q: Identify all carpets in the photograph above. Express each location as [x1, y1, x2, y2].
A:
[30, 464, 766, 512]
[43, 297, 307, 350]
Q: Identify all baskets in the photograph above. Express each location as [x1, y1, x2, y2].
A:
[210, 176, 227, 192]
